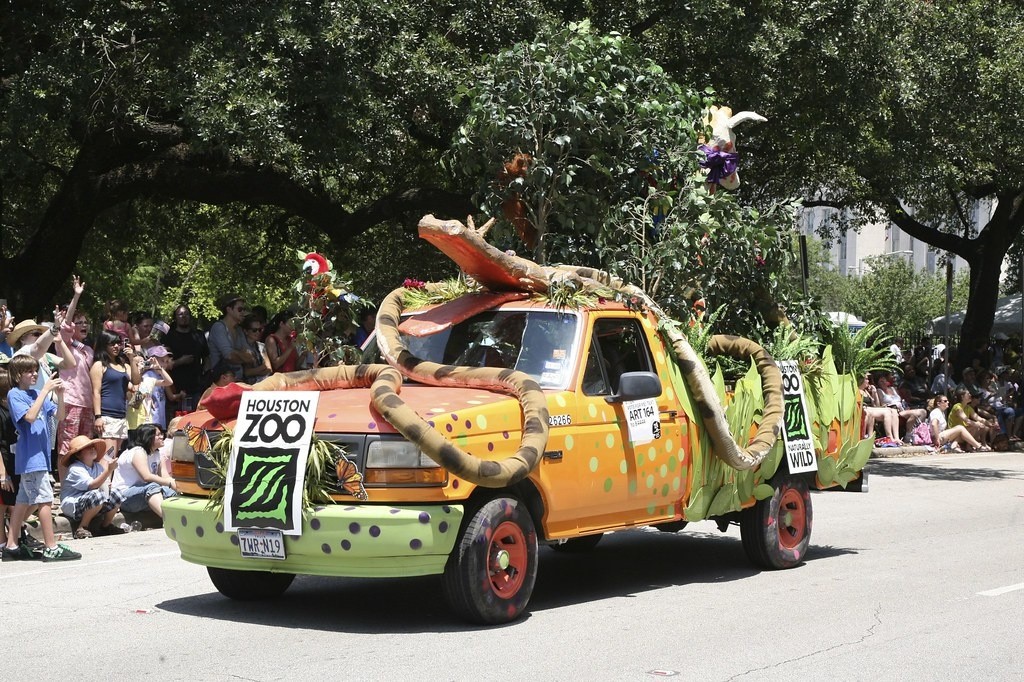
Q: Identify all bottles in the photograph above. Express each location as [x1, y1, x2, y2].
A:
[901, 399, 909, 411]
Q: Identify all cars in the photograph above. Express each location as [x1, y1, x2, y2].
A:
[822, 311, 866, 336]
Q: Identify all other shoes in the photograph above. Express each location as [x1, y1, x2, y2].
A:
[973, 446, 991, 452]
[99, 524, 125, 535]
[74, 527, 92, 538]
[1009, 434, 1023, 442]
[949, 447, 965, 453]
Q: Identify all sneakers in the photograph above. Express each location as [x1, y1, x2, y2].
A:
[6, 540, 32, 554]
[2, 545, 42, 561]
[42, 545, 82, 562]
[21, 533, 44, 550]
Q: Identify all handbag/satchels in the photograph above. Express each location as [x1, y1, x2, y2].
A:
[913, 415, 933, 445]
[260, 336, 282, 374]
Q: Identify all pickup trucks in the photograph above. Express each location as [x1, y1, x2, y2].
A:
[161, 298, 882, 627]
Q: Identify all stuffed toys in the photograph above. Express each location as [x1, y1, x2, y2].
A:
[496, 153, 538, 250]
[295, 249, 378, 315]
[197, 214, 783, 487]
[630, 105, 768, 238]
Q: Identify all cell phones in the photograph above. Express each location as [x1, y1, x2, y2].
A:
[52, 367, 59, 379]
[124, 338, 129, 348]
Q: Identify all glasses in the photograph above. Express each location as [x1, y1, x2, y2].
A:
[108, 341, 122, 346]
[231, 307, 244, 312]
[940, 400, 948, 403]
[155, 433, 164, 437]
[21, 331, 44, 338]
[248, 327, 262, 333]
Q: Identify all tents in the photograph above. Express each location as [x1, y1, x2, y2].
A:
[929, 292, 1023, 335]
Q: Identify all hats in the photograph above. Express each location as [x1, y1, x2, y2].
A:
[147, 346, 172, 359]
[60, 435, 106, 466]
[994, 332, 1010, 340]
[7, 319, 50, 347]
[217, 294, 246, 310]
[962, 367, 974, 376]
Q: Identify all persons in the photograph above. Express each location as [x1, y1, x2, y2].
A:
[562, 345, 613, 397]
[857, 332, 1024, 452]
[458, 318, 517, 370]
[0, 275, 388, 563]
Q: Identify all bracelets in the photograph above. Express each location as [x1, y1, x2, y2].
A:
[42, 389, 49, 394]
[301, 353, 306, 357]
[50, 325, 61, 337]
[0, 476, 11, 482]
[169, 480, 172, 488]
[155, 366, 163, 376]
[95, 415, 101, 420]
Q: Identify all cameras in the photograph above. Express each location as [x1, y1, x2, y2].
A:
[144, 361, 151, 367]
